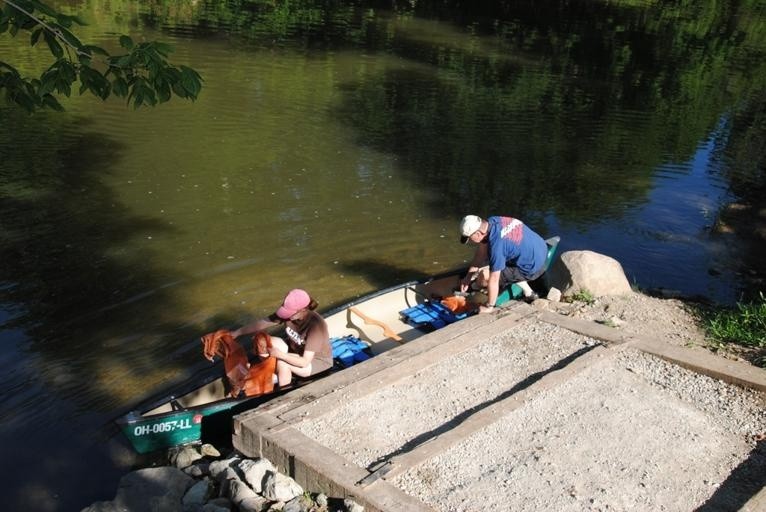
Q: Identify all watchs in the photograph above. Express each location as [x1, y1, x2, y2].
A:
[486, 302, 497, 308]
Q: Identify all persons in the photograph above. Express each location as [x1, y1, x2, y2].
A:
[228, 289, 334, 390]
[460, 215, 548, 314]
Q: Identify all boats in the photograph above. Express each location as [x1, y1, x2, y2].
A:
[121, 226, 569, 455]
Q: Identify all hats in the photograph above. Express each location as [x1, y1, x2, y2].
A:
[276, 288, 311, 319]
[460, 215, 482, 245]
